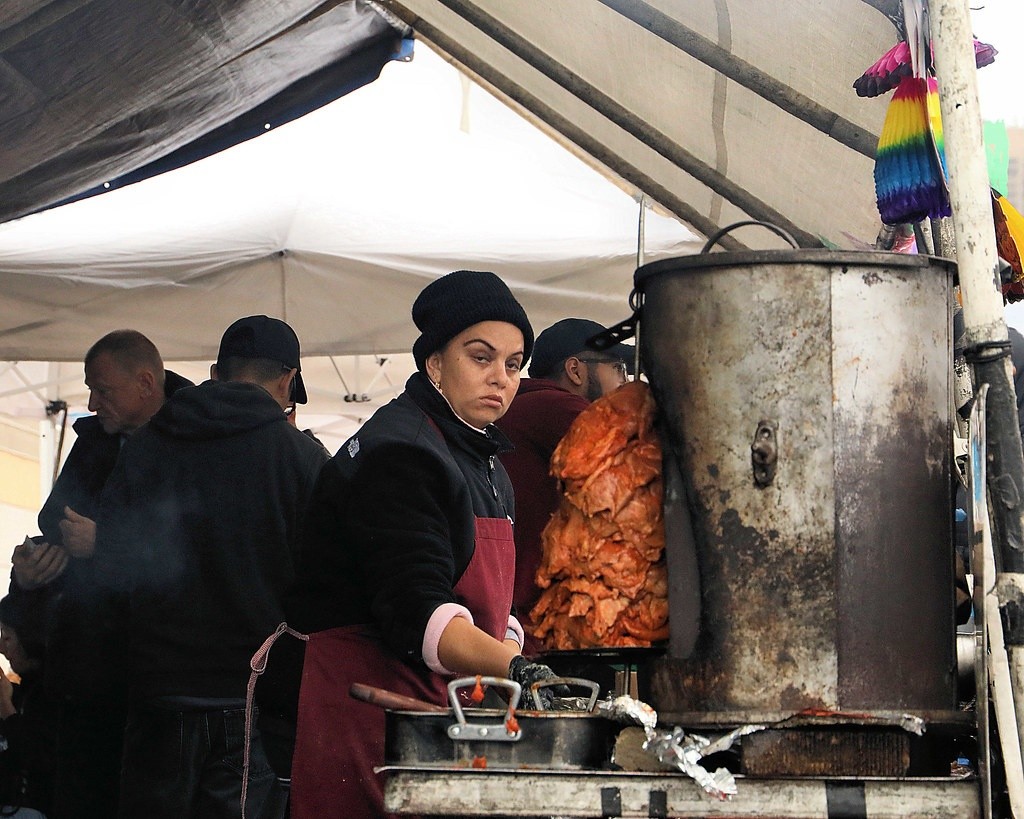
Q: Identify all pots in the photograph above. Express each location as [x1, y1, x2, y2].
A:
[385, 678, 615, 771]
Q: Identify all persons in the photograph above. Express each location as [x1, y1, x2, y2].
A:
[0, 315, 331, 819]
[491, 319, 636, 655]
[240, 271, 585, 819]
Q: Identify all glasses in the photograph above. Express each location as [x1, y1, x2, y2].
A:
[282, 364, 297, 417]
[560, 356, 628, 382]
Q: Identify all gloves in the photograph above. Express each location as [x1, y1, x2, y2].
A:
[508, 654, 570, 713]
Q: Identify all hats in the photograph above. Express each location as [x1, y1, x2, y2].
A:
[219, 314, 307, 404]
[531, 318, 640, 375]
[411, 270, 534, 371]
